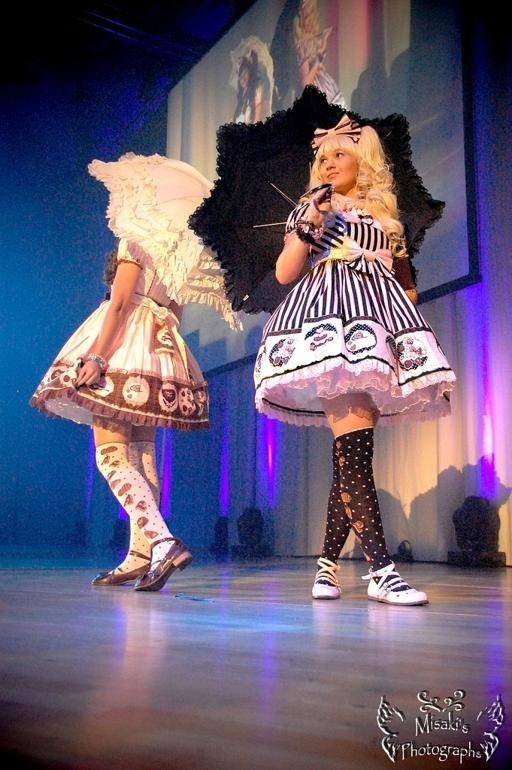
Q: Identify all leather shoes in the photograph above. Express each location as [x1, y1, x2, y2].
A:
[134, 538, 193, 591]
[361, 560, 427, 605]
[312, 557, 341, 598]
[92, 551, 152, 586]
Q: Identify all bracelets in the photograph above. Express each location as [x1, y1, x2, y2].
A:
[87, 354, 109, 371]
[295, 218, 325, 245]
[316, 50, 325, 62]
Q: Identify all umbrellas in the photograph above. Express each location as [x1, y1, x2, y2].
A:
[88, 152, 244, 333]
[184, 80, 445, 314]
[266, 2, 300, 101]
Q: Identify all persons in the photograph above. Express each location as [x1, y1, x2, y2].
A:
[28, 152, 246, 591]
[253, 113, 460, 606]
[79, 586, 167, 769]
[270, 1, 347, 112]
